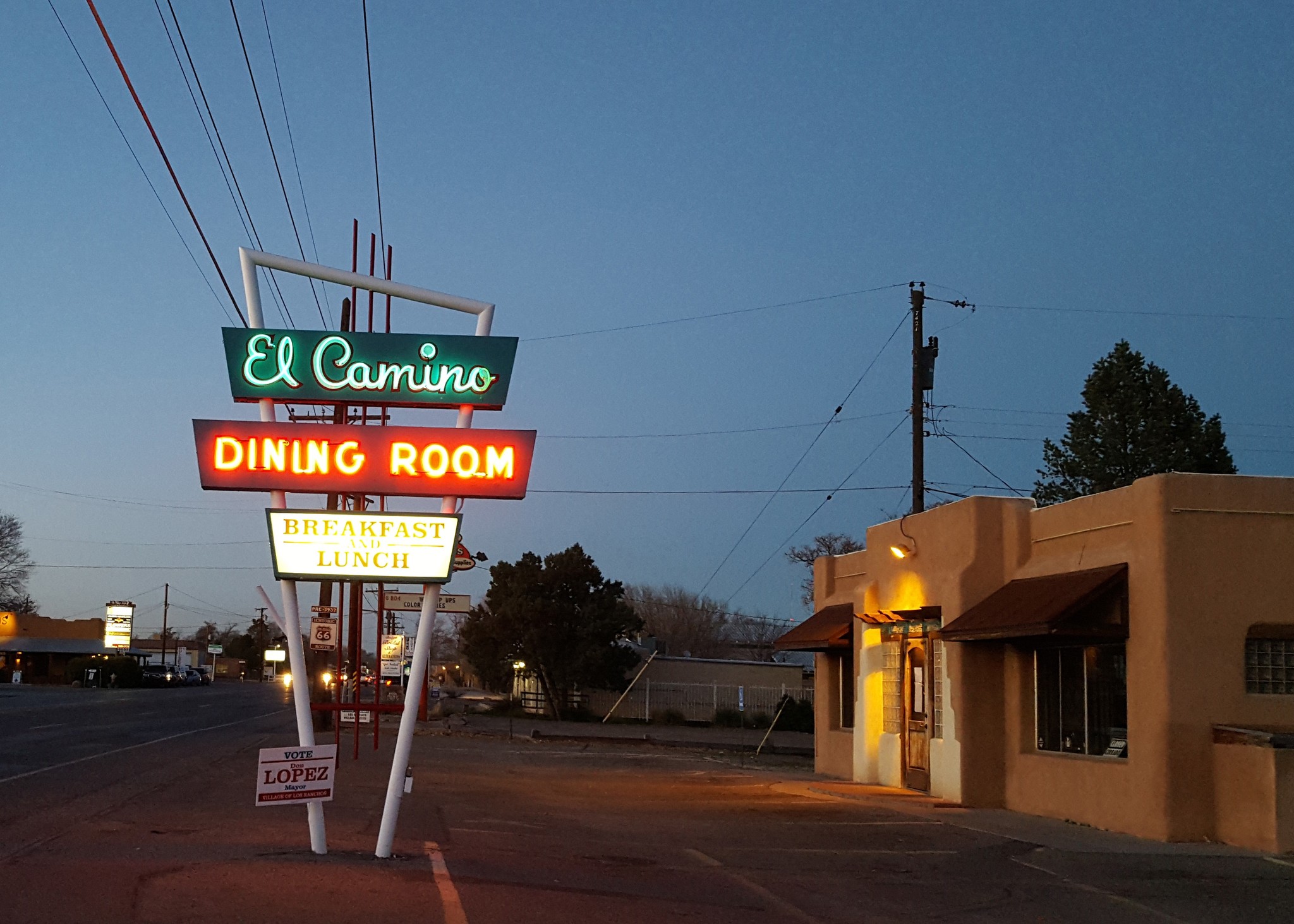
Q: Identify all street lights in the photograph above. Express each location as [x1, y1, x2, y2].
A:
[273, 644, 280, 682]
[513, 661, 526, 697]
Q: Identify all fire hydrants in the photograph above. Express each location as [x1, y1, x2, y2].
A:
[110, 673, 117, 688]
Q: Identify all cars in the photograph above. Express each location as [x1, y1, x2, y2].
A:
[142, 665, 210, 688]
[322, 665, 387, 686]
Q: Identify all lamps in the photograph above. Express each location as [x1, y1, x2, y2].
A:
[890, 543, 917, 559]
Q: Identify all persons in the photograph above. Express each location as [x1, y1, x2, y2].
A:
[433, 689, 469, 734]
